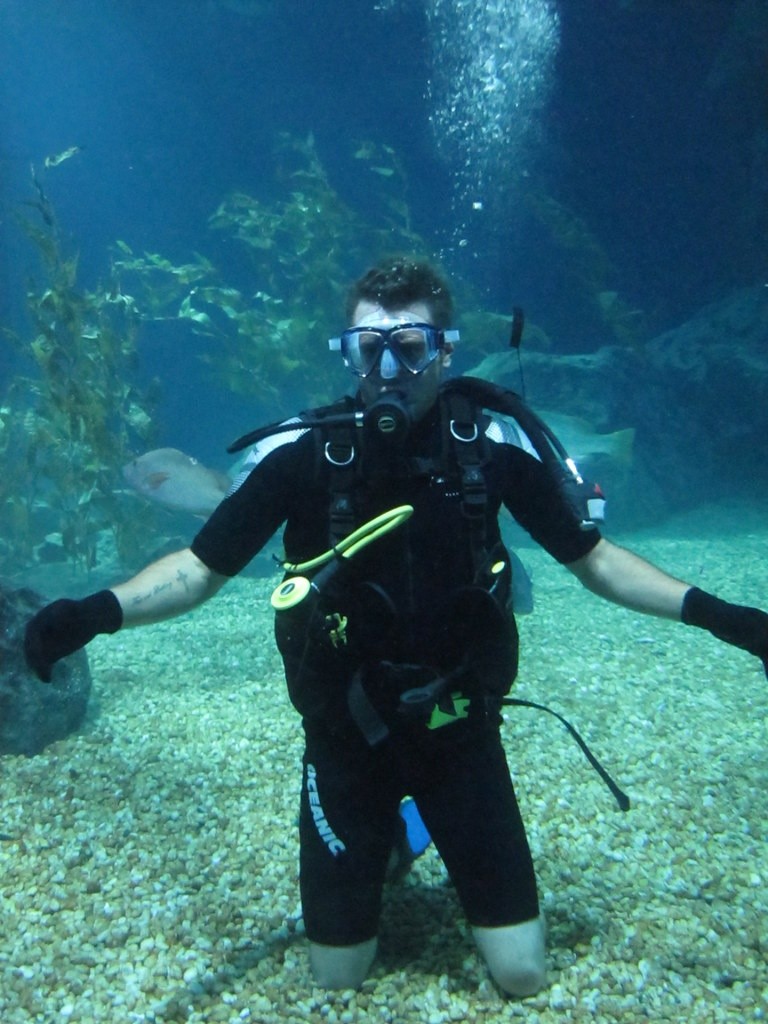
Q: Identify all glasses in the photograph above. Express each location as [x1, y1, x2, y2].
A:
[336, 322, 449, 378]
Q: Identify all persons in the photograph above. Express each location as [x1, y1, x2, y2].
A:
[23, 261, 768, 998]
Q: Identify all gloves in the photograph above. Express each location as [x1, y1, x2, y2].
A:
[680, 585, 768, 676]
[26, 588, 123, 681]
